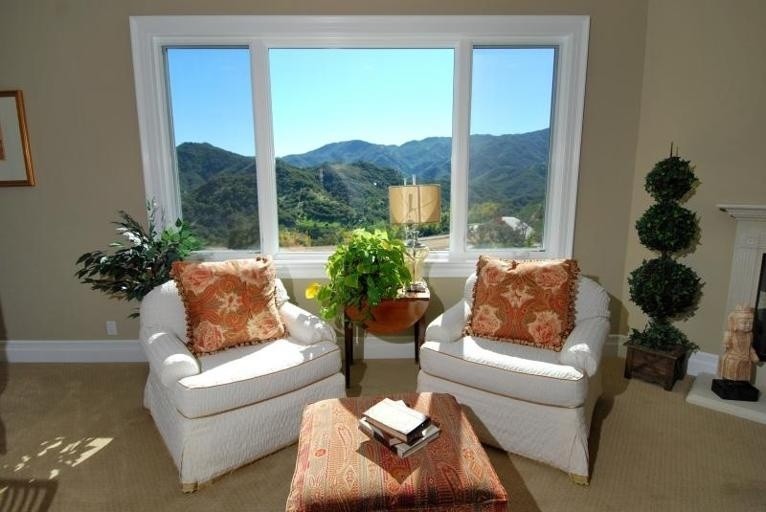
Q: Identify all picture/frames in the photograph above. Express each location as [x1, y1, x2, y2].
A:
[0, 90, 36, 188]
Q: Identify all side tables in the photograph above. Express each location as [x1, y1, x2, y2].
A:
[344, 278, 431, 389]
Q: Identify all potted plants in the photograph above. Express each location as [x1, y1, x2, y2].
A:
[623, 157, 704, 391]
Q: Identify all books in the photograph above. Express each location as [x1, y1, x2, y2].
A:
[359, 398, 440, 459]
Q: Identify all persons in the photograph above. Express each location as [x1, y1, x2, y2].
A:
[721, 305, 759, 381]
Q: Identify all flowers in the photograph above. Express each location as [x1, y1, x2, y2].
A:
[305, 227, 415, 329]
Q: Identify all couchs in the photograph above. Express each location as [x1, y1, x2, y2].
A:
[416, 273, 611, 486]
[139, 279, 347, 492]
[286, 392, 510, 512]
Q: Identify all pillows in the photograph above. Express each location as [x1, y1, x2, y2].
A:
[462, 254, 581, 352]
[172, 254, 291, 357]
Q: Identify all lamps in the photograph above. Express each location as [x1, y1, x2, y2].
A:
[388, 174, 441, 293]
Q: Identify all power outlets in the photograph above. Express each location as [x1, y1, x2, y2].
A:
[105, 320, 118, 336]
